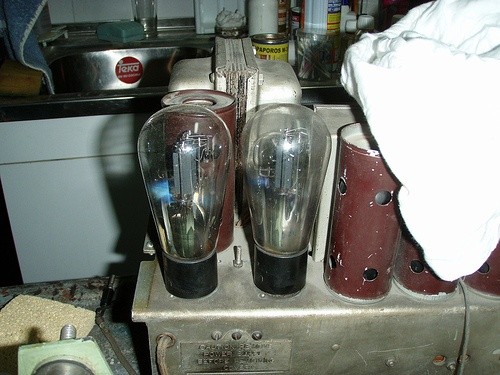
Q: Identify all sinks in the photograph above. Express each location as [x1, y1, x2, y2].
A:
[36, 39, 217, 97]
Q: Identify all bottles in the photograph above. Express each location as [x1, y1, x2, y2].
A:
[342, 14, 375, 55]
[289, 7, 302, 40]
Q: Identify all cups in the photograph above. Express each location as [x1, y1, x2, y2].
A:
[131, 0, 158, 40]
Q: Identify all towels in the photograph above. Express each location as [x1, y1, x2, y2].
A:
[0, 0, 56, 96]
[339, 0, 499, 283]
